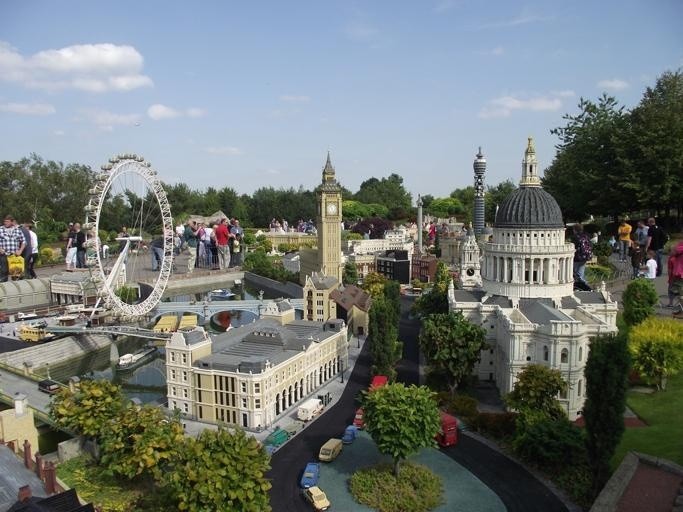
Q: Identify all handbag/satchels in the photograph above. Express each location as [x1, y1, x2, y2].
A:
[7, 254, 25, 274]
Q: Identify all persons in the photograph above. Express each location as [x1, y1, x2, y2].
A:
[271, 218, 313, 233]
[0, 215, 38, 282]
[571, 218, 683, 315]
[66, 222, 101, 269]
[422, 221, 435, 240]
[119, 226, 129, 252]
[441, 223, 449, 237]
[150, 217, 244, 272]
